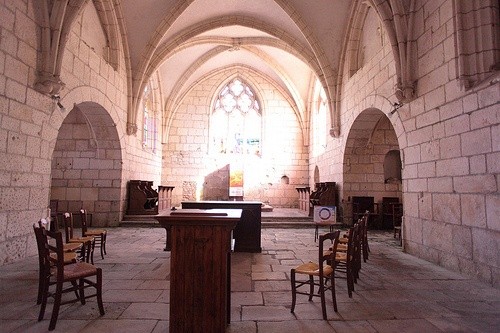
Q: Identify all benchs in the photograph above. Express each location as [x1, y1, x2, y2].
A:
[127, 180, 158, 216]
[309, 182, 335, 216]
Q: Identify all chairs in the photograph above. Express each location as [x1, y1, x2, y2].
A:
[352, 196, 380, 230]
[290, 230, 340, 320]
[382, 197, 403, 240]
[318, 210, 369, 298]
[33, 200, 107, 330]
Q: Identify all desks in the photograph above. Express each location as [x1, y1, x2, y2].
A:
[159, 186, 175, 210]
[180, 202, 262, 252]
[155, 209, 243, 333]
[296, 186, 311, 216]
